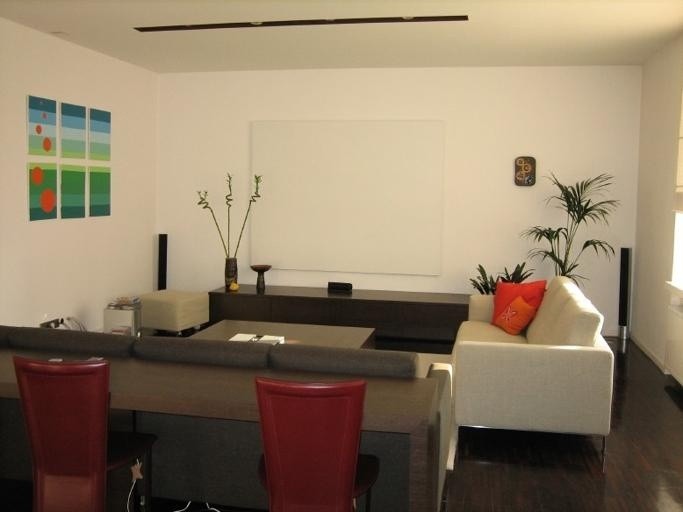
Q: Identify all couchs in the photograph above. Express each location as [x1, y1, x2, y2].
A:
[445, 273, 616, 473]
[136, 288, 210, 333]
[0, 324, 454, 512]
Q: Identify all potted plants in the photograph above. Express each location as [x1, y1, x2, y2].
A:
[198, 172, 262, 292]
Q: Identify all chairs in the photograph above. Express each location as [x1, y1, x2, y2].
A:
[254, 376, 381, 512]
[9, 351, 122, 512]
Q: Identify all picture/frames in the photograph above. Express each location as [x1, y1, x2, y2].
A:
[28, 161, 57, 222]
[25, 93, 59, 159]
[58, 163, 86, 220]
[87, 165, 111, 217]
[87, 107, 111, 162]
[57, 102, 88, 162]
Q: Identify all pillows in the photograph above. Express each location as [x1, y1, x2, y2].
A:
[490, 278, 549, 337]
